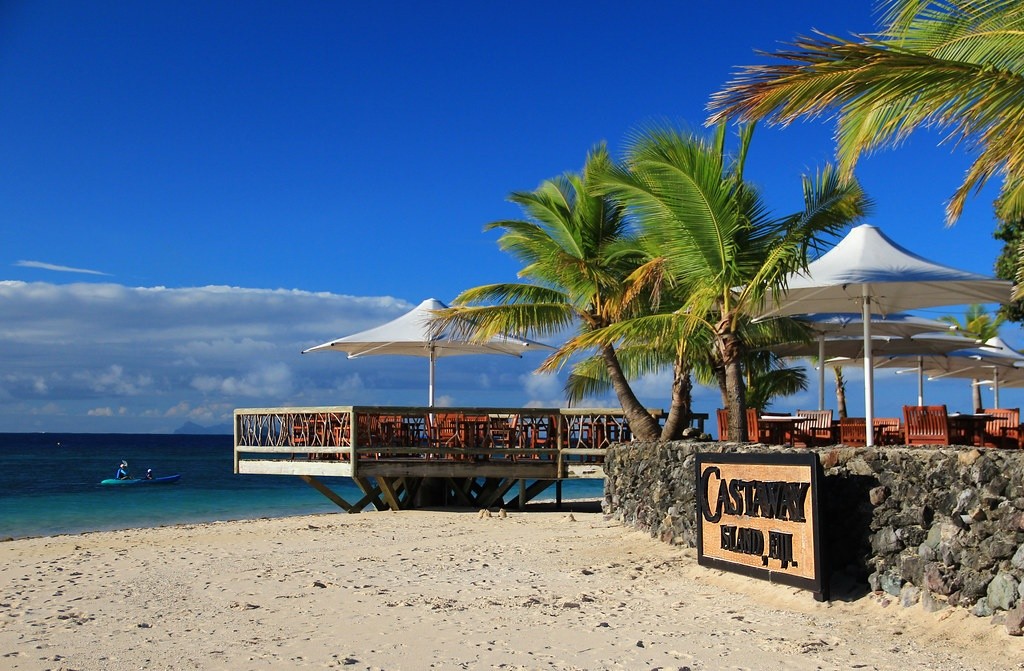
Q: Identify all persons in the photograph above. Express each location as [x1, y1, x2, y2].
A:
[117, 463, 134, 480]
[143, 469, 154, 480]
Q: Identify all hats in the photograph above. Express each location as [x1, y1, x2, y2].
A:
[148, 469, 151, 473]
[120, 464, 124, 467]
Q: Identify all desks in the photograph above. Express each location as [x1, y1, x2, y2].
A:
[758, 418, 817, 447]
[835, 424, 897, 446]
[948, 415, 1008, 447]
[574, 422, 627, 463]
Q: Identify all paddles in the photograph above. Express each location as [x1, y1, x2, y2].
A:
[145, 465, 153, 480]
[122, 459, 134, 479]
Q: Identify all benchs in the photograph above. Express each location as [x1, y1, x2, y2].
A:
[716, 404, 1024, 449]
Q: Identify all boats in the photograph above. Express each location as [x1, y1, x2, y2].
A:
[101, 474, 182, 488]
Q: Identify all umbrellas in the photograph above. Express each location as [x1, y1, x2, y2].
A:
[301, 297, 559, 456]
[675, 222, 1024, 448]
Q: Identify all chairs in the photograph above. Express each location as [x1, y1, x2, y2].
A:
[292, 414, 572, 461]
